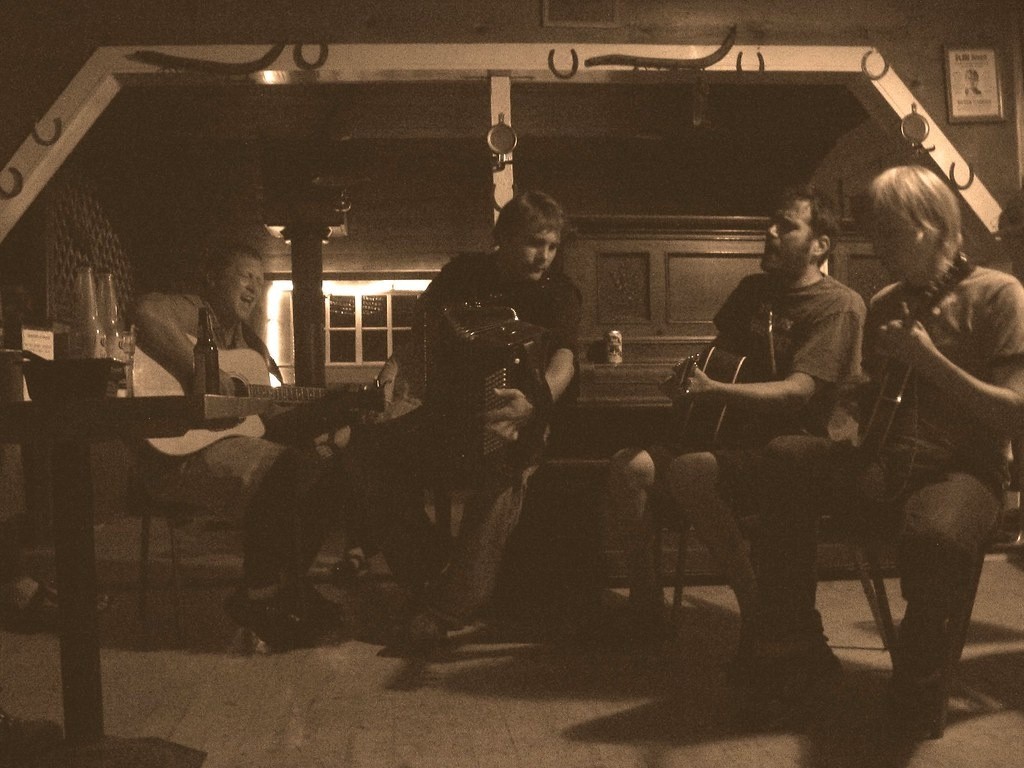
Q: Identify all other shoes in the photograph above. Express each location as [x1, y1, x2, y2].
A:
[281, 582, 343, 620]
[224, 590, 310, 648]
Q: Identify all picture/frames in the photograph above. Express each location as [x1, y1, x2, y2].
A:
[542, 0, 618, 28]
[942, 40, 1005, 126]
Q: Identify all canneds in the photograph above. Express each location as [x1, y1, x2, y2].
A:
[605, 330, 623, 364]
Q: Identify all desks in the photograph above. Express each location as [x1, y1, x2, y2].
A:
[1, 395, 271, 768]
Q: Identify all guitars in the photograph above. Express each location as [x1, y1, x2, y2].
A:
[824, 250, 973, 515]
[666, 345, 753, 447]
[128, 329, 390, 457]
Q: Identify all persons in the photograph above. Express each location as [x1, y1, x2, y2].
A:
[334, 188, 584, 647]
[129, 245, 344, 641]
[748, 162, 1024, 739]
[593, 186, 866, 686]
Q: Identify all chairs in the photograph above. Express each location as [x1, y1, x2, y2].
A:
[128, 465, 239, 639]
[369, 433, 1023, 727]
